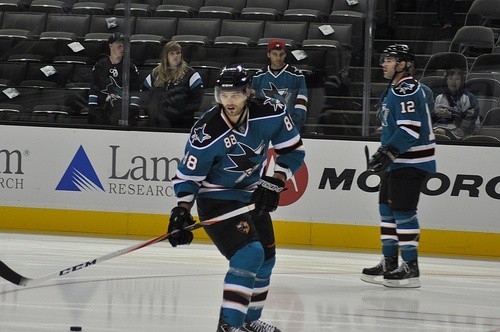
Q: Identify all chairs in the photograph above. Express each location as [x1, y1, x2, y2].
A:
[0, 0, 500, 146]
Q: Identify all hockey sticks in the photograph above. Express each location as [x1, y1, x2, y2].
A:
[0, 204, 255, 287]
[364, 144, 371, 170]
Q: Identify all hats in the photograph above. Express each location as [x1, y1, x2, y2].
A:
[109, 33, 125, 43]
[268, 39, 286, 49]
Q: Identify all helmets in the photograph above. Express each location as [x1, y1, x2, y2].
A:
[383, 44, 415, 59]
[216, 66, 253, 97]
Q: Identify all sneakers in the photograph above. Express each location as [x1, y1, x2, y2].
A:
[243, 319, 281, 332]
[361, 257, 398, 284]
[384, 260, 421, 287]
[216, 319, 252, 332]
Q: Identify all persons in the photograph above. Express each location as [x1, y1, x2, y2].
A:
[250, 40, 308, 139]
[167, 64, 305, 332]
[360, 44, 437, 289]
[432, 67, 480, 146]
[142, 41, 204, 129]
[88, 33, 142, 127]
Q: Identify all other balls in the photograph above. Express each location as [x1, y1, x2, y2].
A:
[70, 325, 82, 331]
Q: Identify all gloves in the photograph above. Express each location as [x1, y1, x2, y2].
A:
[367, 145, 400, 173]
[167, 207, 193, 247]
[250, 176, 288, 217]
